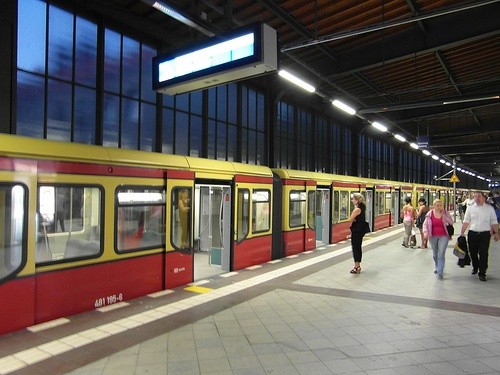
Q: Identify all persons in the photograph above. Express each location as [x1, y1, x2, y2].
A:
[422, 199, 454, 280]
[416, 197, 430, 248]
[455, 191, 499, 234]
[460, 191, 500, 281]
[57, 189, 66, 234]
[178, 191, 191, 251]
[400, 197, 415, 248]
[349, 192, 366, 274]
[81, 193, 92, 228]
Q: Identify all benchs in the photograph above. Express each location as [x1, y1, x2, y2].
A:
[63, 239, 99, 258]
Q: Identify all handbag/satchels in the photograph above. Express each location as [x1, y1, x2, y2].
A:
[447, 224, 454, 237]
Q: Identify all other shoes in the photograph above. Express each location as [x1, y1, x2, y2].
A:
[472, 271, 477, 274]
[479, 275, 486, 281]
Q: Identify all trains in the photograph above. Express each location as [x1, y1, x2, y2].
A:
[0, 133, 492, 334]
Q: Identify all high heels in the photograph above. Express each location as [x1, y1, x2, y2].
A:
[351, 267, 361, 273]
